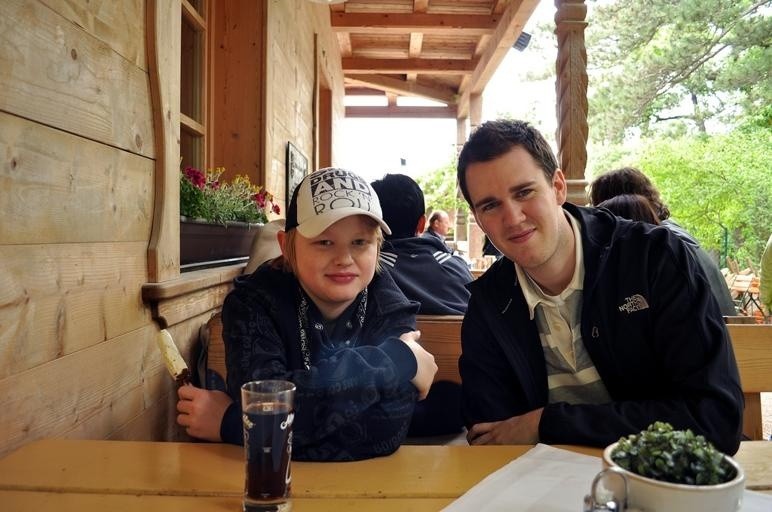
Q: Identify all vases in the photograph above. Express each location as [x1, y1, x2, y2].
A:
[180, 214, 266, 272]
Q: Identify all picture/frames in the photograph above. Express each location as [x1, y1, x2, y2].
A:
[286, 141, 309, 210]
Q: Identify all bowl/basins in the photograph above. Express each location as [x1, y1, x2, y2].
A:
[601, 440, 744, 511]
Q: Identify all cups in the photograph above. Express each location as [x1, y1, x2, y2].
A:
[240, 380, 297, 512]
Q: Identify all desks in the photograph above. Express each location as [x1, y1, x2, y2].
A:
[0, 432, 770, 511]
[732, 287, 766, 317]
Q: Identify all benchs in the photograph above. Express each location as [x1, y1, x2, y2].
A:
[196, 314, 771, 446]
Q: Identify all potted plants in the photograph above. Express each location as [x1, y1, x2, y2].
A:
[602, 419, 748, 512]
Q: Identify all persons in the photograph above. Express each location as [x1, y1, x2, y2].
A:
[424, 211, 453, 256]
[760, 235, 772, 314]
[592, 168, 737, 315]
[177, 168, 438, 463]
[458, 120, 743, 457]
[370, 174, 476, 315]
[592, 194, 660, 225]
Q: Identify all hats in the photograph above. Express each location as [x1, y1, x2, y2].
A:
[284, 166, 393, 238]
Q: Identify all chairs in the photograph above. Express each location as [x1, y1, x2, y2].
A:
[729, 274, 755, 315]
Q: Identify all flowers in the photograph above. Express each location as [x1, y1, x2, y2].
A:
[180, 165, 281, 230]
